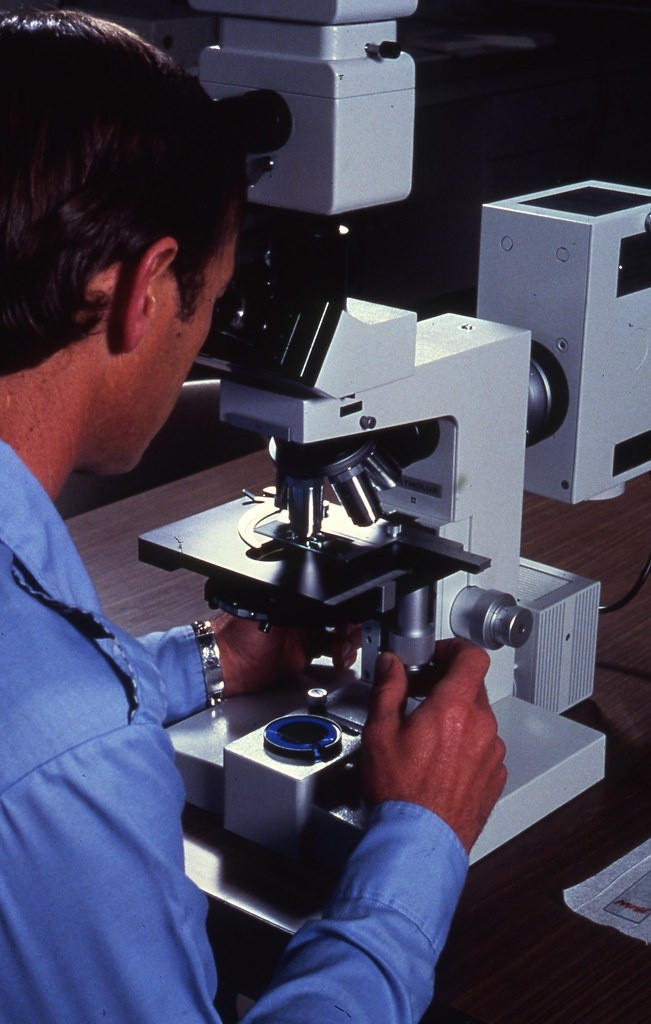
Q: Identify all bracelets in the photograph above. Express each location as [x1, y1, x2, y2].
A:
[191, 618, 225, 705]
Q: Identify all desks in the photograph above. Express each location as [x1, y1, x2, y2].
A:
[63, 445, 651, 1024]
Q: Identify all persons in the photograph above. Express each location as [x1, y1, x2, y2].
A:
[0, 11, 503, 1024]
